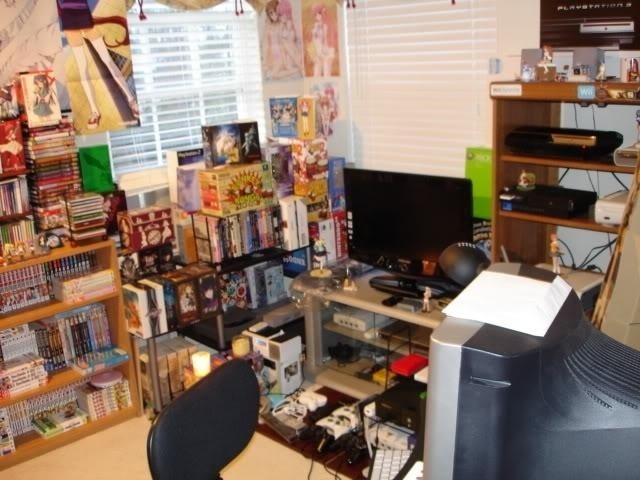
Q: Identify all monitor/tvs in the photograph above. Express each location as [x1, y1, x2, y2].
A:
[422, 262, 640, 480]
[343, 167, 474, 299]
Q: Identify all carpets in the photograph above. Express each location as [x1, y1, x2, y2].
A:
[257, 386, 374, 480]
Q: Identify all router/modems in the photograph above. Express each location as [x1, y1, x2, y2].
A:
[333, 307, 390, 332]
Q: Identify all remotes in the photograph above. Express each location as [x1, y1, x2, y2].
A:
[274, 412, 307, 432]
[261, 412, 298, 443]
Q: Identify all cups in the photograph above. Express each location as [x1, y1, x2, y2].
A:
[619, 56, 640, 84]
[192, 351, 211, 378]
[231, 334, 251, 357]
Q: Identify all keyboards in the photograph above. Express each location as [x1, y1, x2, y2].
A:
[371, 449, 412, 480]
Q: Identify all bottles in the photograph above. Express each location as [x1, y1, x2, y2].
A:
[520, 64, 532, 83]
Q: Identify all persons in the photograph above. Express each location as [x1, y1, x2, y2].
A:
[596, 59, 608, 87]
[423, 284, 431, 314]
[261, 3, 302, 78]
[548, 233, 563, 276]
[55, 1, 139, 129]
[542, 45, 553, 74]
[305, 4, 339, 78]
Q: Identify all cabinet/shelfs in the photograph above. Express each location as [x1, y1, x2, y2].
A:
[131, 311, 225, 414]
[1, 240, 140, 475]
[211, 243, 311, 351]
[293, 258, 458, 401]
[490, 80, 640, 266]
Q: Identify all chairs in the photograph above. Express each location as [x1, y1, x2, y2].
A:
[147, 357, 260, 479]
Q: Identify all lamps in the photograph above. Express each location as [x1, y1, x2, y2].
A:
[437, 242, 491, 286]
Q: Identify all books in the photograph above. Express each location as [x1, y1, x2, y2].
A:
[116, 195, 347, 409]
[0, 71, 134, 455]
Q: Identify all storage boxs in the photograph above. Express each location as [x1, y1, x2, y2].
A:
[197, 162, 277, 217]
[242, 321, 303, 394]
[115, 207, 176, 252]
